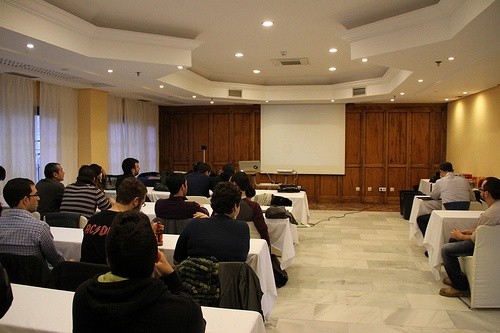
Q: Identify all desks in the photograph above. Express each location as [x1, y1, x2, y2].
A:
[399, 179, 486, 283]
[0, 187, 311, 333]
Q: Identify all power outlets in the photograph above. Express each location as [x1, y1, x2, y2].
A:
[356, 186, 360, 192]
[390, 186, 395, 192]
[378, 187, 387, 191]
[367, 186, 373, 192]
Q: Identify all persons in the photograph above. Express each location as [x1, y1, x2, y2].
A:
[82, 158, 239, 197]
[32, 164, 111, 227]
[172, 181, 252, 265]
[439, 177, 500, 297]
[79, 176, 151, 264]
[72, 210, 207, 333]
[210, 172, 273, 252]
[417, 162, 476, 258]
[0, 262, 14, 319]
[154, 175, 208, 222]
[0, 177, 75, 286]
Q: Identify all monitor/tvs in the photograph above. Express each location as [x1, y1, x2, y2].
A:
[239, 161, 260, 174]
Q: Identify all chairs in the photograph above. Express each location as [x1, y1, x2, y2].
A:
[48, 261, 110, 293]
[1, 208, 41, 220]
[0, 252, 51, 289]
[441, 199, 483, 211]
[172, 262, 265, 322]
[43, 212, 89, 229]
[186, 194, 211, 204]
[102, 175, 124, 190]
[457, 225, 500, 310]
[137, 172, 162, 187]
[160, 218, 193, 235]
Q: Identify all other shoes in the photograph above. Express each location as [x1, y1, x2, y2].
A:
[443, 278, 452, 285]
[440, 286, 469, 297]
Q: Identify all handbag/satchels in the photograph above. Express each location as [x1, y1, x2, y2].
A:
[266, 207, 287, 219]
[271, 254, 288, 287]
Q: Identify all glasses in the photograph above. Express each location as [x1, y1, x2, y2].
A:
[141, 201, 146, 207]
[479, 188, 489, 192]
[21, 193, 39, 199]
